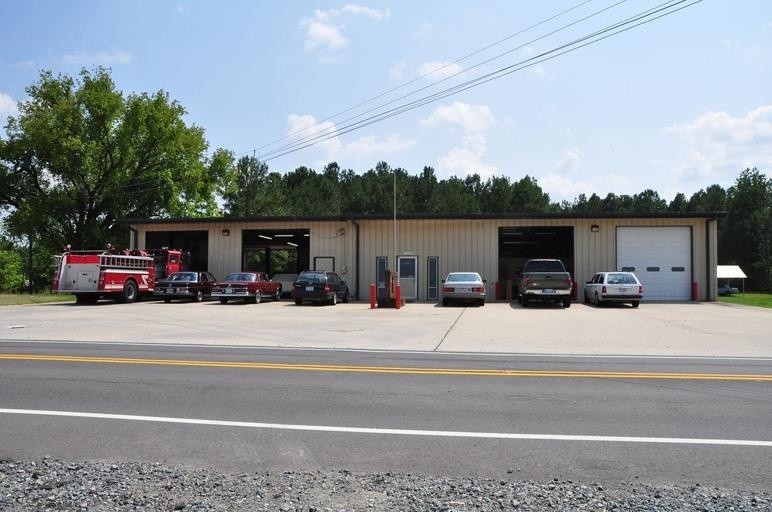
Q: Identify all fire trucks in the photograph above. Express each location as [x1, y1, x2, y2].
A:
[50, 243, 191, 302]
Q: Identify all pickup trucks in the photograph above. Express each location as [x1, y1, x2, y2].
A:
[516, 258, 573, 307]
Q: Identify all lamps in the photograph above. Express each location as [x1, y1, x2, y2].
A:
[591, 225, 599, 232]
[222, 229, 230, 236]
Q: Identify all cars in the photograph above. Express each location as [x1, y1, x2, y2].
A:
[441, 272, 486, 305]
[291, 270, 349, 304]
[584, 272, 644, 307]
[718, 282, 739, 297]
[152, 272, 217, 302]
[211, 271, 282, 304]
